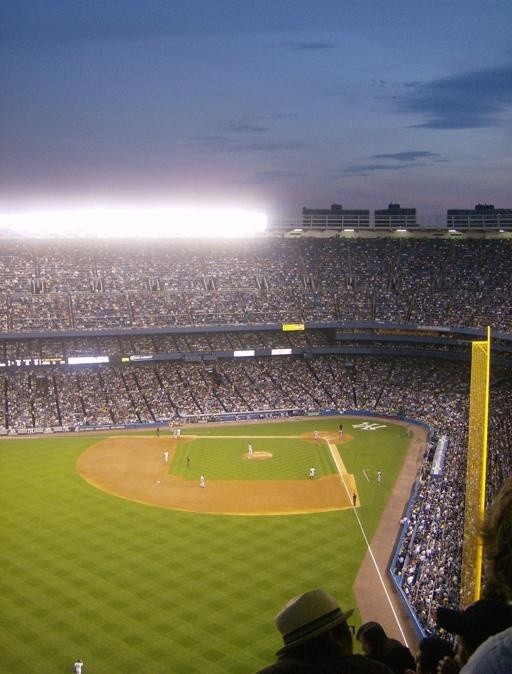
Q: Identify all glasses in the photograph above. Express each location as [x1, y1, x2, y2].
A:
[345, 625, 356, 637]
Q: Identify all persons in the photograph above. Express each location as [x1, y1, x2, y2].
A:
[256, 587, 393, 672]
[403, 598, 512, 673]
[309, 466, 316, 479]
[392, 475, 511, 643]
[168, 418, 183, 439]
[200, 474, 206, 488]
[376, 469, 382, 481]
[356, 622, 416, 672]
[353, 493, 356, 506]
[74, 659, 83, 674]
[163, 447, 169, 464]
[247, 441, 254, 456]
[187, 454, 192, 467]
[337, 423, 343, 437]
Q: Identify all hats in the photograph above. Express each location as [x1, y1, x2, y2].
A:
[436, 600, 510, 636]
[274, 588, 355, 657]
